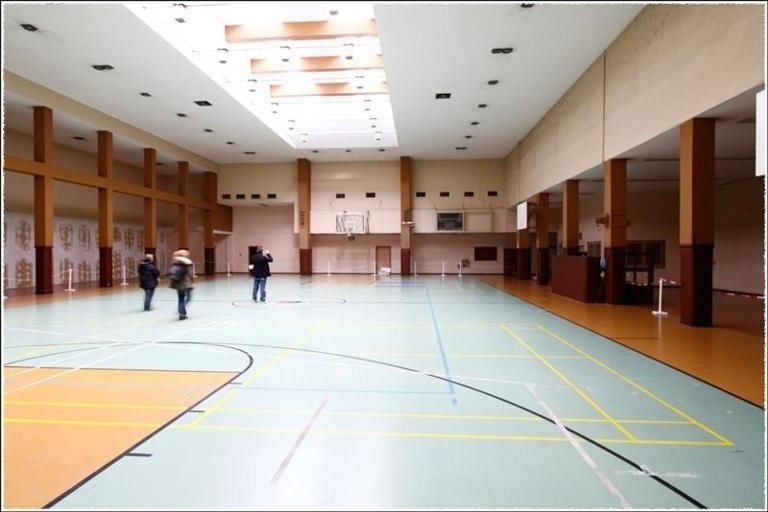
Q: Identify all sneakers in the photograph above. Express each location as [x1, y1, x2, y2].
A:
[252, 296, 266, 303]
[179, 313, 188, 320]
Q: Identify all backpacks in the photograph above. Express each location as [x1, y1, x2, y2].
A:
[166, 258, 187, 281]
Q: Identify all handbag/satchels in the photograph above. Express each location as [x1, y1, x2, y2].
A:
[248, 263, 254, 277]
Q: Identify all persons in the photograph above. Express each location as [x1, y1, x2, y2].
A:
[249, 246, 273, 303]
[137, 253, 160, 310]
[168, 249, 194, 320]
